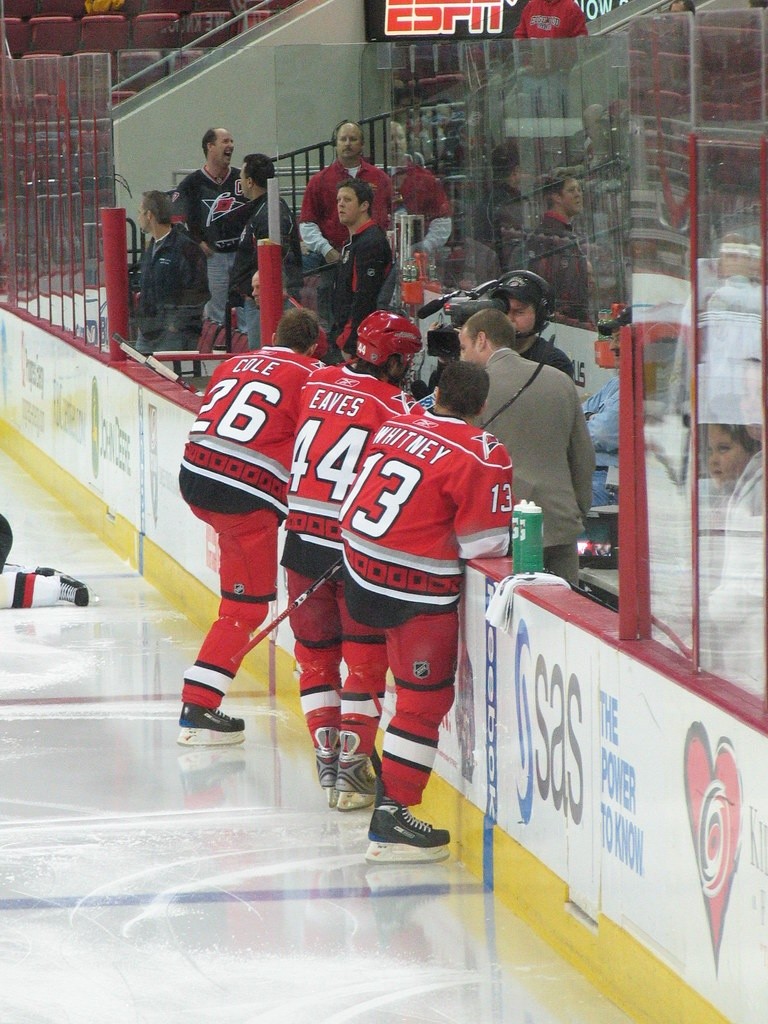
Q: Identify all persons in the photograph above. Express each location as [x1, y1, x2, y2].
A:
[426, 269, 574, 392]
[0, 513, 98, 610]
[337, 361, 522, 867]
[238, 268, 296, 351]
[436, 310, 593, 591]
[657, 229, 765, 701]
[296, 120, 394, 291]
[126, 190, 211, 351]
[326, 180, 393, 355]
[170, 128, 253, 325]
[174, 310, 327, 750]
[278, 310, 426, 807]
[576, 310, 631, 508]
[513, 0, 588, 117]
[383, 71, 628, 329]
[668, 0, 696, 22]
[227, 153, 301, 306]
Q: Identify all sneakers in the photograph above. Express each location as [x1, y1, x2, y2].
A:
[36, 566, 96, 607]
[179, 703, 246, 746]
[364, 780, 452, 865]
[312, 726, 341, 806]
[333, 731, 377, 809]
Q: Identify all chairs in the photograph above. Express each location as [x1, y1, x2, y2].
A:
[1, 1, 291, 295]
[390, 13, 761, 263]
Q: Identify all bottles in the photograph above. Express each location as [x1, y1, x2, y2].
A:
[401, 260, 417, 283]
[598, 303, 626, 341]
[512, 499, 543, 574]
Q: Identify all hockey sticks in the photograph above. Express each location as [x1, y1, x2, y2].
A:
[110, 332, 205, 397]
[228, 334, 559, 665]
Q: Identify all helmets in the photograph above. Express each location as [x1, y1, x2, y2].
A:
[488, 271, 552, 333]
[356, 311, 422, 366]
